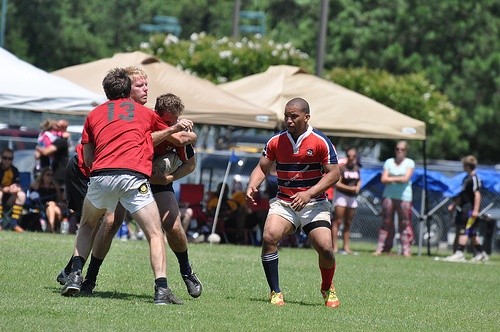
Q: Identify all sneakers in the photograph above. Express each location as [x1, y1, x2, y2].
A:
[320, 287, 341, 308]
[269, 291, 285, 306]
[60, 272, 84, 297]
[152, 286, 183, 305]
[179, 269, 203, 298]
[81, 277, 96, 292]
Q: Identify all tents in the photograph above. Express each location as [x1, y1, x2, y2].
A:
[0, 48, 108, 111]
[357, 164, 500, 255]
[217, 65, 431, 255]
[44, 51, 278, 131]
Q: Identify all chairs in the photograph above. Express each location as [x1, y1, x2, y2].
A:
[179, 183, 268, 244]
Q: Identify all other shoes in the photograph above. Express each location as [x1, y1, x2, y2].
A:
[444, 251, 466, 262]
[57, 261, 74, 283]
[469, 251, 490, 264]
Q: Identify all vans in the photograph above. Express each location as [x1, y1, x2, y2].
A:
[196, 152, 269, 193]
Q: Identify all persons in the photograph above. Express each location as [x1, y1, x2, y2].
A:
[330, 146, 362, 255]
[245, 98, 341, 308]
[0, 63, 202, 306]
[444, 154, 489, 264]
[477, 188, 496, 254]
[374, 141, 416, 257]
[178, 207, 193, 231]
[209, 181, 251, 245]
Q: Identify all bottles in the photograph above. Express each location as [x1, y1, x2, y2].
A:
[60, 218, 69, 234]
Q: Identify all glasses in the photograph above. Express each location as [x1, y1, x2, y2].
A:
[3, 156, 13, 161]
[396, 147, 406, 152]
[45, 173, 54, 177]
